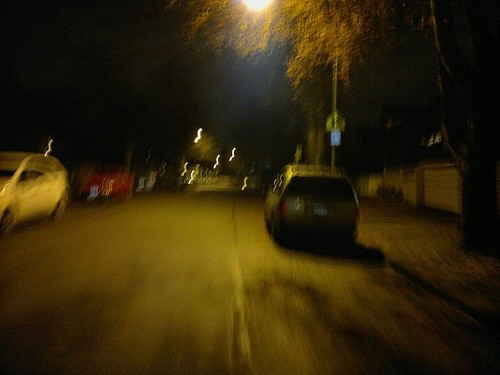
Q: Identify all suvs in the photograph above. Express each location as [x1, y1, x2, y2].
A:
[0, 153, 71, 233]
[263, 163, 363, 249]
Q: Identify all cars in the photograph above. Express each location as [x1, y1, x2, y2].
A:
[74, 158, 170, 203]
